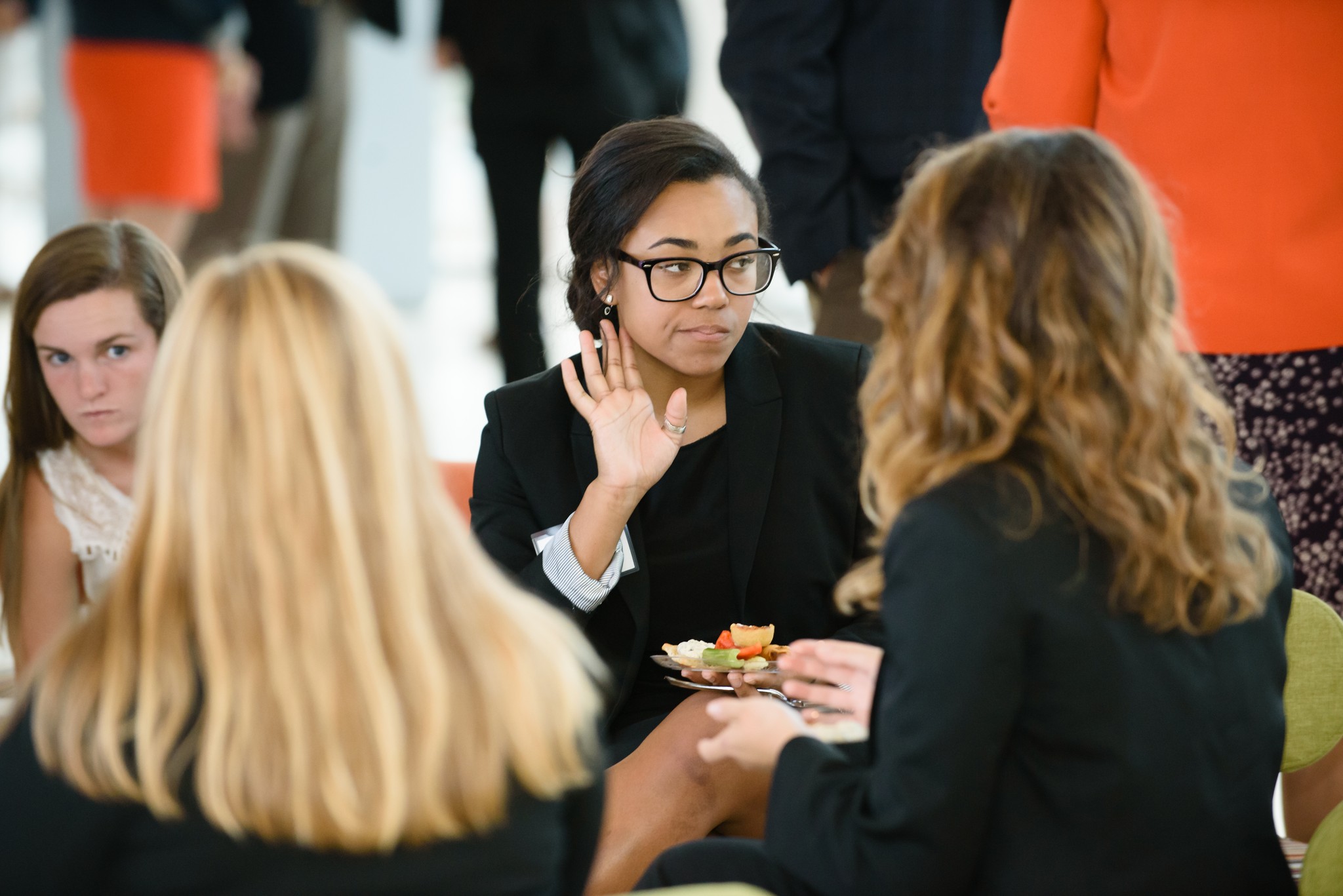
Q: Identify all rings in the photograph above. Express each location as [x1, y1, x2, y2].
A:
[664, 414, 687, 434]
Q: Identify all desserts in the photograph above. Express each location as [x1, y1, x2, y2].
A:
[730, 623, 775, 647]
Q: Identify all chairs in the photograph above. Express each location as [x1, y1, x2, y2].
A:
[1283, 579, 1343, 785]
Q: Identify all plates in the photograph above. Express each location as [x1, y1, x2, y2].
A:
[650, 654, 781, 674]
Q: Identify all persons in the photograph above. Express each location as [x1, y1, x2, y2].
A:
[0, 0, 1341, 896]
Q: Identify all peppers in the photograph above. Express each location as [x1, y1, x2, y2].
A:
[714, 629, 763, 659]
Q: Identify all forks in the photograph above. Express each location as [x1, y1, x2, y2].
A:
[665, 675, 852, 708]
